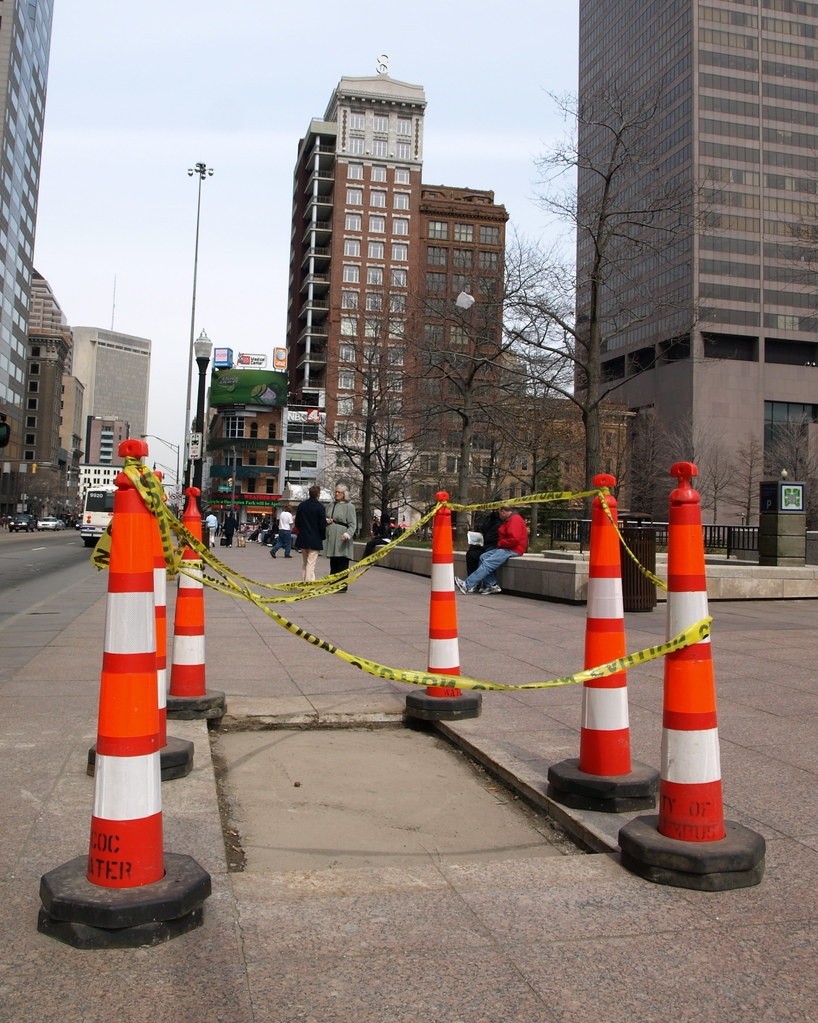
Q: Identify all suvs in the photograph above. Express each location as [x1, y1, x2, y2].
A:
[8, 514, 36, 532]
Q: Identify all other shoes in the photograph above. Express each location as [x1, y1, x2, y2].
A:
[285, 555, 291, 558]
[479, 587, 484, 592]
[468, 587, 475, 592]
[270, 551, 276, 558]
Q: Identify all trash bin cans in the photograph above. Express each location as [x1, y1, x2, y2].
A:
[261, 530, 269, 543]
[202, 520, 210, 551]
[616, 513, 660, 612]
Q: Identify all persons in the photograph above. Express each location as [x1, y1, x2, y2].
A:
[324, 484, 356, 593]
[394, 524, 403, 539]
[224, 512, 239, 548]
[466, 495, 503, 591]
[361, 513, 391, 565]
[270, 506, 294, 559]
[454, 506, 528, 594]
[525, 518, 531, 535]
[206, 510, 218, 549]
[272, 519, 279, 534]
[373, 517, 380, 537]
[295, 485, 326, 581]
[245, 513, 269, 543]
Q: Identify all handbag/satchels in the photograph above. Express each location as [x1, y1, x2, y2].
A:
[467, 531, 484, 546]
[221, 533, 228, 545]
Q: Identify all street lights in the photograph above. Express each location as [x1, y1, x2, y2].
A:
[141, 434, 179, 519]
[181, 162, 215, 511]
[288, 462, 293, 482]
[741, 512, 745, 525]
[193, 329, 213, 516]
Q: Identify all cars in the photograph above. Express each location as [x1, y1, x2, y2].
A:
[75, 519, 83, 530]
[37, 517, 66, 531]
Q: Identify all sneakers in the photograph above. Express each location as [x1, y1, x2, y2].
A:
[455, 576, 468, 595]
[481, 584, 502, 594]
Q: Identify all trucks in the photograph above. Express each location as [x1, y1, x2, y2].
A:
[78, 488, 118, 546]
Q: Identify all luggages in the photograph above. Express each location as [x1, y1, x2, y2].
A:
[252, 530, 258, 541]
[237, 530, 246, 547]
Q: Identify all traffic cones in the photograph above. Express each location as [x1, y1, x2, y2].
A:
[166, 487, 227, 720]
[548, 474, 661, 813]
[38, 440, 212, 950]
[406, 491, 482, 721]
[619, 461, 768, 891]
